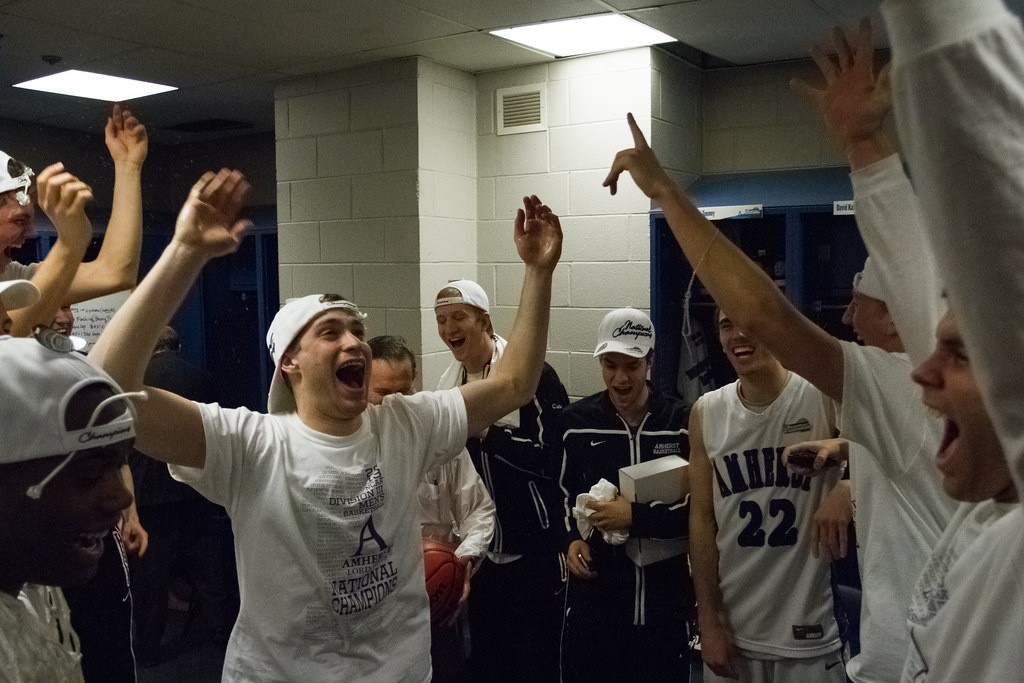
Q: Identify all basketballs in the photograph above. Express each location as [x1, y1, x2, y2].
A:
[422, 536, 464, 624]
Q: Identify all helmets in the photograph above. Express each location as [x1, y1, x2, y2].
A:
[267, 295, 368, 416]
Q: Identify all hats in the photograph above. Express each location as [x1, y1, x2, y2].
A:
[0, 332, 149, 498]
[853, 257, 884, 301]
[434, 280, 494, 336]
[593, 306, 656, 358]
[0, 280, 39, 313]
[0, 151, 36, 205]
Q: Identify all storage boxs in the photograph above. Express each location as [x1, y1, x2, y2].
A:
[618, 455, 690, 569]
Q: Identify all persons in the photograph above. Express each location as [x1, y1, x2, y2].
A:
[50, 303, 149, 683]
[788, 0, 1024, 683]
[132, 325, 213, 667]
[0, 334, 137, 683]
[688, 306, 853, 683]
[434, 278, 571, 683]
[89, 168, 564, 683]
[603, 113, 963, 683]
[552, 305, 693, 683]
[0, 104, 148, 335]
[367, 335, 497, 627]
